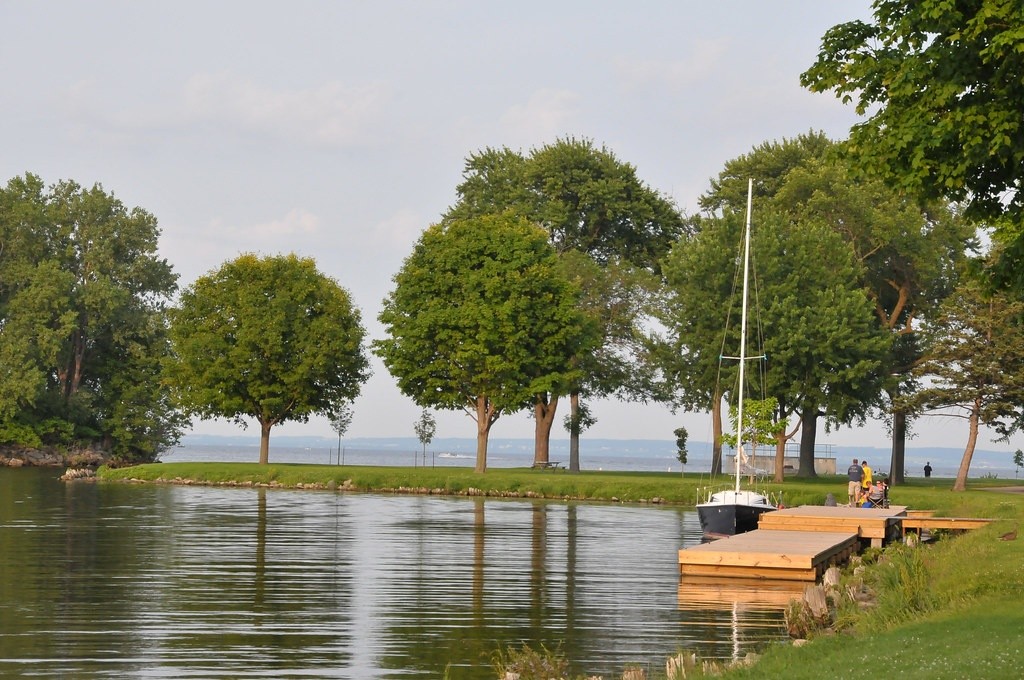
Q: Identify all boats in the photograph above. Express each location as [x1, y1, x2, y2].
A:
[722, 440, 838, 478]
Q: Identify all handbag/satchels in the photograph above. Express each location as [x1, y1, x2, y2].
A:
[862, 502, 872, 508]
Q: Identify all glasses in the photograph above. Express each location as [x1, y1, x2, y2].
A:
[876, 483, 881, 484]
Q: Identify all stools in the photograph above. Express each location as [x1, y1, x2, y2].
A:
[868, 498, 883, 509]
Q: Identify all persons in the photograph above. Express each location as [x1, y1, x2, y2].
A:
[860, 461, 872, 504]
[924, 462, 932, 478]
[847, 459, 865, 507]
[857, 478, 888, 508]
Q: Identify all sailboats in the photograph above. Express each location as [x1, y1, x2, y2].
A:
[694, 177, 789, 542]
